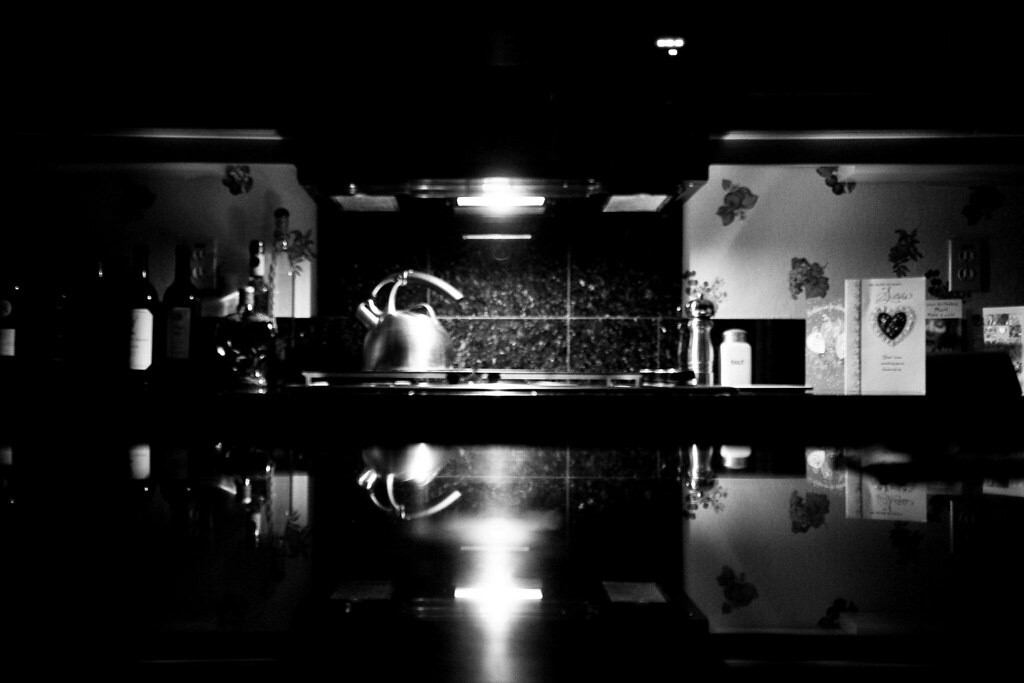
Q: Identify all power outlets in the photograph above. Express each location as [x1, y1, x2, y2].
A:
[948, 239, 984, 292]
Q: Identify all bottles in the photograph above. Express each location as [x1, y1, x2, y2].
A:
[128, 443, 290, 549]
[269, 210, 316, 318]
[719, 329, 752, 386]
[0, 278, 21, 367]
[27, 240, 278, 396]
[720, 445, 752, 470]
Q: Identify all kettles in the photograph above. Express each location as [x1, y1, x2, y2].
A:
[354, 271, 464, 371]
[357, 443, 462, 521]
